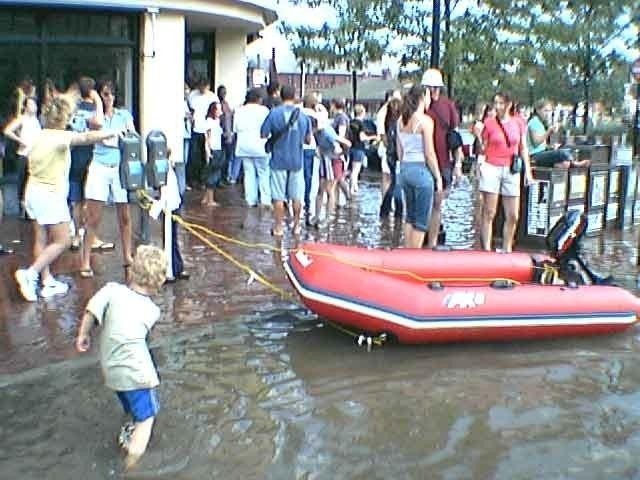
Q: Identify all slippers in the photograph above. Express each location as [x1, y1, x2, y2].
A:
[79, 268, 95, 278]
[90, 240, 115, 249]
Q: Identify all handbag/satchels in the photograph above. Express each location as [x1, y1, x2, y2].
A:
[264, 110, 299, 152]
[495, 114, 524, 175]
[435, 113, 463, 150]
[212, 150, 227, 169]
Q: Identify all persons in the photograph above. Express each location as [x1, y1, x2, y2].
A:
[3, 98, 42, 220]
[199, 101, 226, 207]
[302, 94, 316, 227]
[259, 84, 311, 236]
[12, 97, 139, 303]
[379, 98, 403, 218]
[225, 150, 242, 185]
[78, 79, 134, 278]
[396, 84, 442, 249]
[527, 100, 592, 170]
[39, 78, 57, 128]
[329, 95, 352, 206]
[421, 68, 459, 249]
[472, 90, 535, 254]
[349, 104, 378, 195]
[307, 115, 352, 229]
[11, 74, 38, 113]
[69, 74, 116, 250]
[516, 102, 525, 117]
[217, 84, 236, 184]
[450, 146, 464, 179]
[233, 87, 270, 208]
[184, 82, 194, 192]
[148, 148, 190, 283]
[376, 88, 395, 211]
[187, 78, 227, 190]
[473, 101, 487, 209]
[74, 244, 170, 469]
[262, 82, 282, 108]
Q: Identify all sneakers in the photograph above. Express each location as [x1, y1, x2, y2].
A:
[14, 269, 38, 302]
[40, 277, 70, 298]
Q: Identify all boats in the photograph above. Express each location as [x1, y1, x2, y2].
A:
[282, 209, 637, 346]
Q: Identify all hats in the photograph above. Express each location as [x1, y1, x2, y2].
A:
[421, 67, 444, 87]
